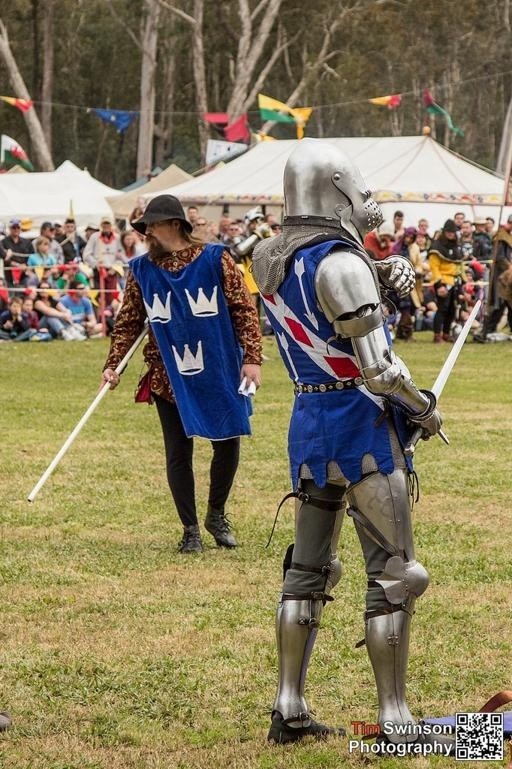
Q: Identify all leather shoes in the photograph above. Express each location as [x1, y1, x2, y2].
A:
[267, 714, 347, 745]
[433, 333, 447, 342]
[375, 720, 458, 756]
[443, 332, 455, 343]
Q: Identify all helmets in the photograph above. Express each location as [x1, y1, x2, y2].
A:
[287, 137, 381, 243]
[244, 205, 265, 235]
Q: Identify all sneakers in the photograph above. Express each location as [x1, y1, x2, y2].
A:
[178, 525, 202, 554]
[204, 510, 238, 547]
[28, 328, 89, 342]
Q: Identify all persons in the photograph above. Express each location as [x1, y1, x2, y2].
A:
[250, 137, 444, 753]
[101, 194, 263, 553]
[1, 203, 512, 343]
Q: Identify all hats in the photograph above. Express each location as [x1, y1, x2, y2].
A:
[85, 223, 99, 231]
[471, 216, 489, 224]
[379, 220, 395, 237]
[440, 220, 461, 231]
[101, 216, 111, 224]
[8, 218, 21, 229]
[41, 222, 57, 230]
[19, 216, 32, 228]
[131, 194, 193, 233]
